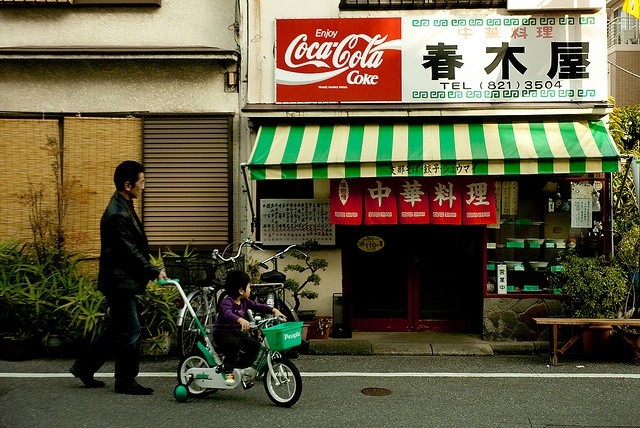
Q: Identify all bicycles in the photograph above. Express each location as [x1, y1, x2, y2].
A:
[175, 238, 263, 359]
[258, 244, 308, 326]
[157, 278, 303, 407]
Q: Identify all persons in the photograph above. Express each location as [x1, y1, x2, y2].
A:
[212, 270, 287, 385]
[68, 160, 170, 394]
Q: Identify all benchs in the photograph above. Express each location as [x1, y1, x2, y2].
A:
[533, 317, 640, 366]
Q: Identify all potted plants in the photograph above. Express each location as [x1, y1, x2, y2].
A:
[280, 240, 328, 342]
[0, 236, 105, 362]
[545, 254, 630, 353]
[135, 281, 181, 357]
[616, 225, 640, 359]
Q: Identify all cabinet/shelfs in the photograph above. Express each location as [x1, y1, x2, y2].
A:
[483, 218, 612, 296]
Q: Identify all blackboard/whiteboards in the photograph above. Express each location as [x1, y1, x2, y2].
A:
[260, 199, 335, 245]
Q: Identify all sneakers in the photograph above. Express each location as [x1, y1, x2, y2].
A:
[223, 373, 236, 385]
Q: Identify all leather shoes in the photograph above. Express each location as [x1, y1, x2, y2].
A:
[69, 364, 106, 387]
[114, 379, 155, 396]
